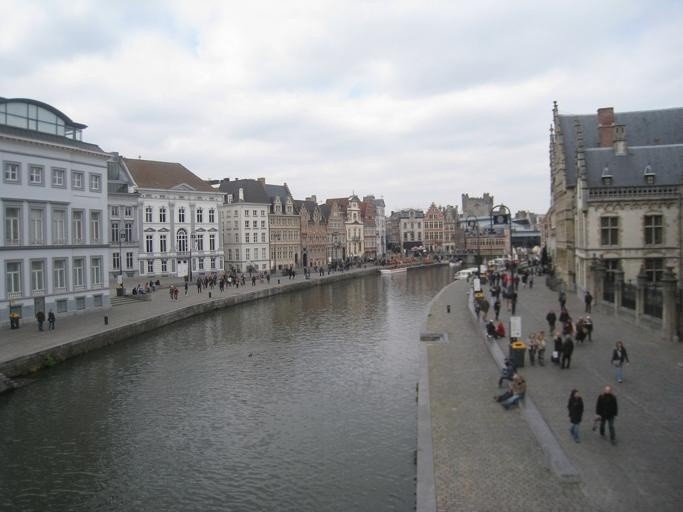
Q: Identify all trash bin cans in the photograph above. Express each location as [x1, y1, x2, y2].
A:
[479, 272, 487, 285]
[475, 293, 484, 310]
[508, 342, 528, 368]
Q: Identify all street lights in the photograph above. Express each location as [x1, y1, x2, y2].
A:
[463, 214, 481, 292]
[117, 229, 126, 288]
[488, 204, 515, 345]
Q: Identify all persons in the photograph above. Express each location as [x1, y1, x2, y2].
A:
[132, 280, 160, 295]
[266, 273, 271, 284]
[169, 271, 256, 300]
[288, 269, 296, 279]
[319, 261, 348, 277]
[303, 266, 311, 277]
[474, 258, 631, 444]
[36, 309, 55, 331]
[10, 313, 20, 330]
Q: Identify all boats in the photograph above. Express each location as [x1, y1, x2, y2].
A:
[378, 267, 407, 274]
[448, 259, 462, 268]
[381, 272, 406, 277]
[453, 267, 477, 280]
